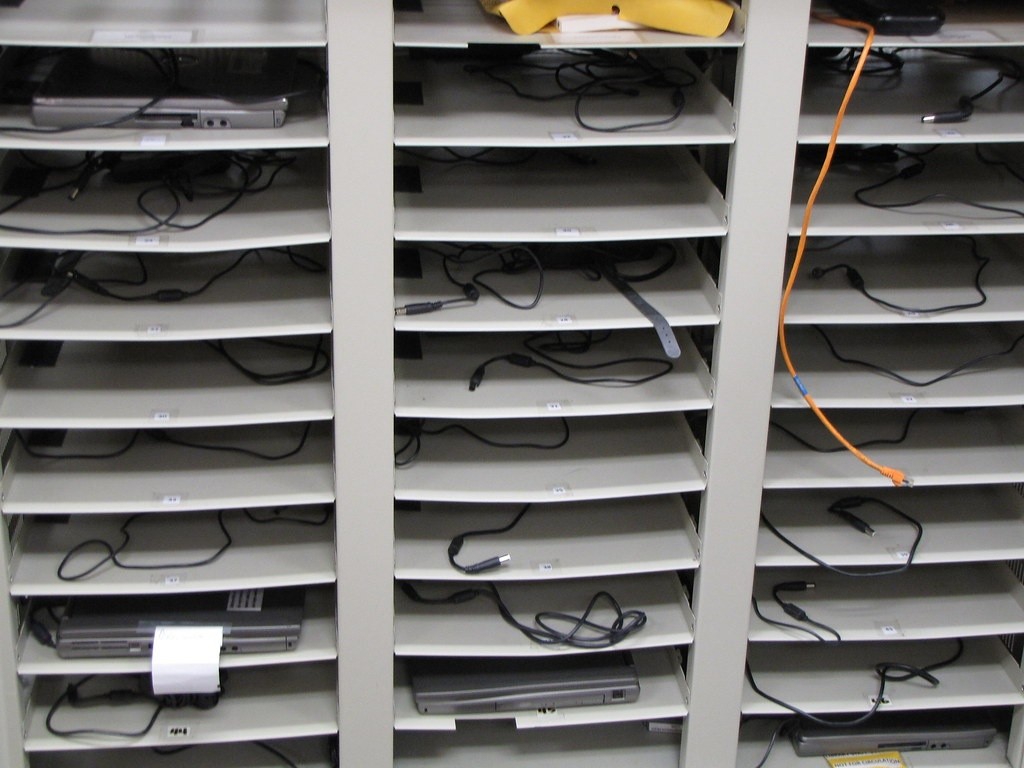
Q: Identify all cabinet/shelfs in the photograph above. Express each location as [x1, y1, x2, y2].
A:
[1, 1, 1024, 768]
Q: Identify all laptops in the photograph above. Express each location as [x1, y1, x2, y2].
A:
[402, 650, 639, 715]
[57, 586, 304, 660]
[786, 705, 999, 757]
[30, 69, 290, 129]
